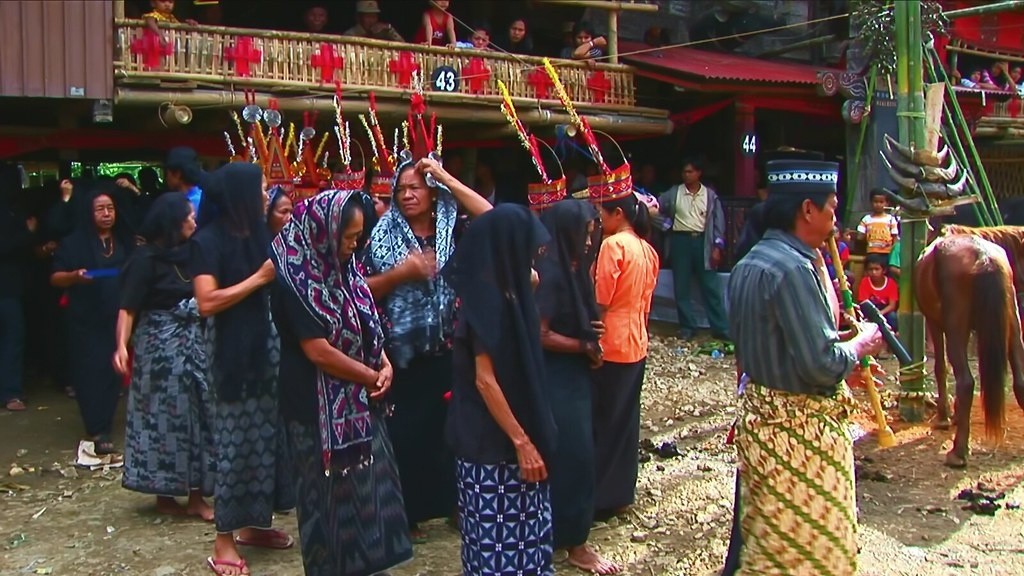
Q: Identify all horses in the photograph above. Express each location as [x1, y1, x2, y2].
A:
[914, 224, 1024, 467]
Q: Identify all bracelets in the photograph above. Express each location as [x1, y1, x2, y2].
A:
[589, 40, 594, 47]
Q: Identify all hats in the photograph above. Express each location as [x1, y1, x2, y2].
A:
[150, 148, 198, 170]
[357, 1, 380, 13]
[766, 159, 840, 195]
[223, 58, 633, 203]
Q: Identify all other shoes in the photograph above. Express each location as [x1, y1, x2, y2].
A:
[879, 348, 891, 361]
[677, 326, 693, 340]
[65, 385, 77, 397]
[715, 332, 734, 343]
[410, 530, 426, 543]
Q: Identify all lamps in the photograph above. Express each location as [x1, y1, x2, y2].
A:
[156, 103, 194, 128]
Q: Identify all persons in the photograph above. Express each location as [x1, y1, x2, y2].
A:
[951, 61, 1024, 94]
[658, 158, 731, 342]
[728, 159, 882, 576]
[265, 155, 495, 576]
[437, 203, 554, 576]
[47, 187, 130, 467]
[0, 148, 206, 414]
[587, 164, 658, 518]
[538, 198, 620, 576]
[144, 0, 606, 64]
[820, 184, 899, 335]
[111, 191, 216, 521]
[185, 162, 296, 576]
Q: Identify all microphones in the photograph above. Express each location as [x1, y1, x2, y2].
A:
[859, 299, 912, 366]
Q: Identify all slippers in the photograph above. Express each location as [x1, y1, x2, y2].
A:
[6, 398, 26, 411]
[89, 434, 117, 453]
[235, 527, 294, 549]
[207, 550, 250, 576]
